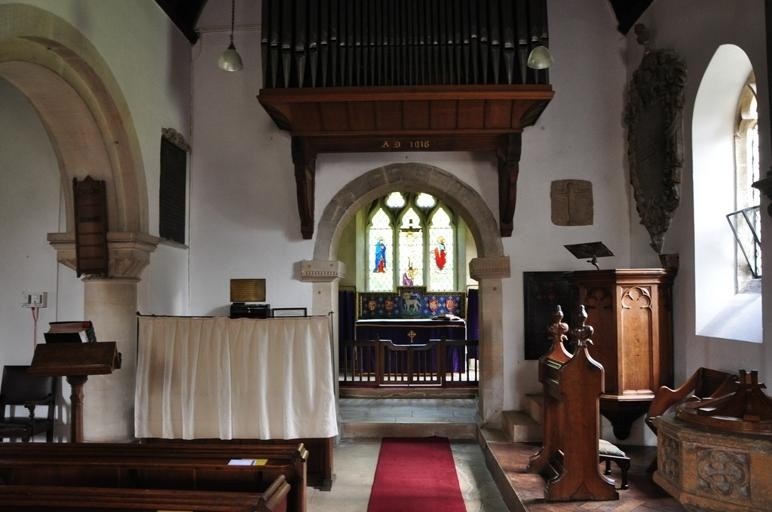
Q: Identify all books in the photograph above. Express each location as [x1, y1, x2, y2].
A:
[44, 321, 96, 344]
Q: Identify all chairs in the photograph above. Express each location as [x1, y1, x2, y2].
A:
[598, 438, 630, 490]
[0, 365, 57, 444]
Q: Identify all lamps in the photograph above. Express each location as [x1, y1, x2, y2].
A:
[217, 0, 244, 72]
[526, 0, 555, 70]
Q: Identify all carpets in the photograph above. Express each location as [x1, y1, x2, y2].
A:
[367, 437, 467, 512]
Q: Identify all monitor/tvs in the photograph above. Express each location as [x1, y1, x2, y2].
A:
[231, 279, 265, 302]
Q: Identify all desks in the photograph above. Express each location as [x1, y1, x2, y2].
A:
[0, 441, 310, 512]
